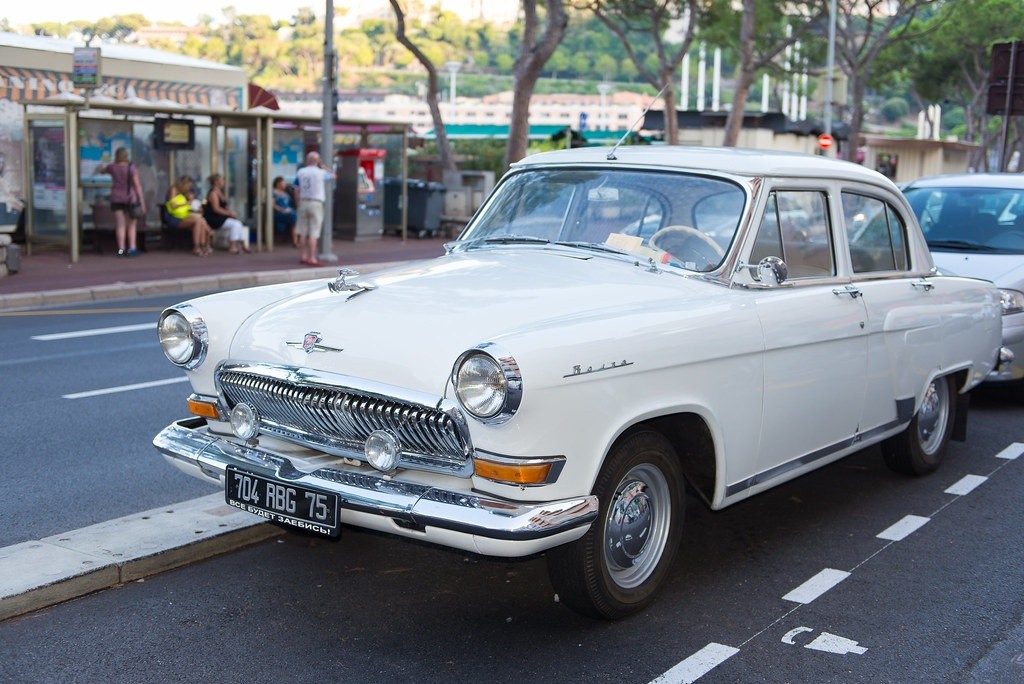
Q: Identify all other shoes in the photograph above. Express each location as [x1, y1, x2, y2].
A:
[243, 246, 252, 253]
[127, 248, 138, 256]
[118, 249, 126, 257]
[300, 258, 308, 264]
[194, 244, 212, 257]
[306, 258, 325, 266]
[228, 247, 239, 254]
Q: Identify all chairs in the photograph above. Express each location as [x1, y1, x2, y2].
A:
[929, 211, 999, 241]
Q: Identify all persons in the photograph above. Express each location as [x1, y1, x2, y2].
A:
[203, 174, 255, 255]
[160, 175, 214, 258]
[98, 147, 147, 256]
[272, 177, 303, 249]
[296, 153, 338, 263]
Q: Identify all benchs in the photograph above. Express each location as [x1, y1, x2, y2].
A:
[662, 235, 874, 279]
[136, 226, 195, 251]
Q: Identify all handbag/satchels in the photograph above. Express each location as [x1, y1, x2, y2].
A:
[130, 202, 142, 219]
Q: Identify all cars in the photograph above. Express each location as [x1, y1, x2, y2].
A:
[154, 144, 1017, 631]
[849, 173, 1024, 383]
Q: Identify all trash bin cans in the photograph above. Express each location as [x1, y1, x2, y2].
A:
[444, 184, 471, 220]
[384, 177, 447, 238]
[470, 187, 484, 215]
